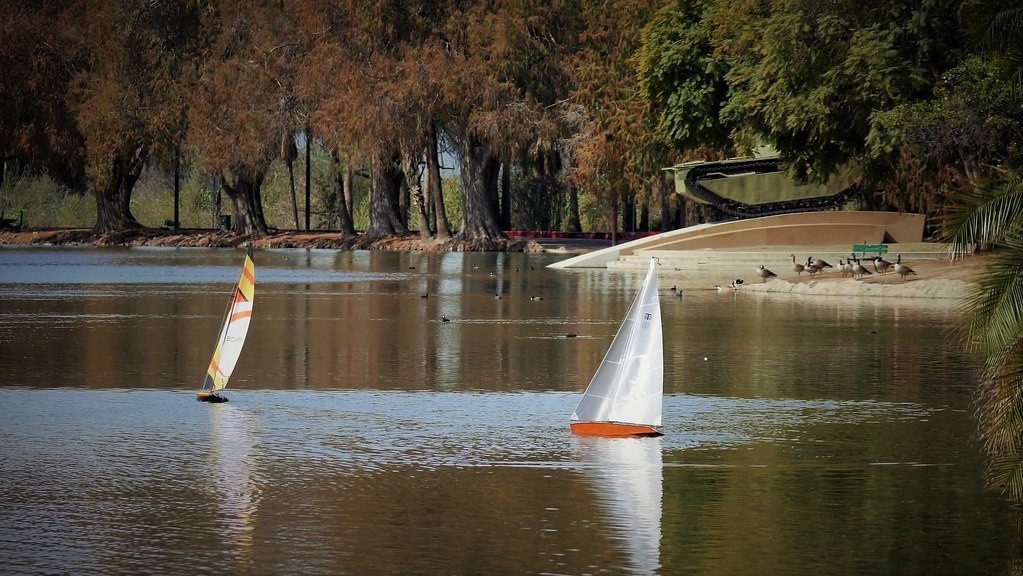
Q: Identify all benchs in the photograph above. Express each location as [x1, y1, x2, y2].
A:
[846, 240, 888, 261]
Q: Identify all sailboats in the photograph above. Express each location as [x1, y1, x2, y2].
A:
[569, 256, 666, 439]
[197, 241, 255, 403]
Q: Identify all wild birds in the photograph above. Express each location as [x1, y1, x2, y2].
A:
[495, 293, 502, 300]
[671, 279, 743, 298]
[409, 267, 416, 269]
[789, 253, 833, 278]
[530, 296, 543, 301]
[757, 265, 777, 283]
[874, 253, 897, 275]
[472, 264, 533, 278]
[839, 253, 873, 280]
[282, 256, 289, 260]
[442, 315, 450, 322]
[421, 294, 428, 298]
[894, 253, 918, 282]
